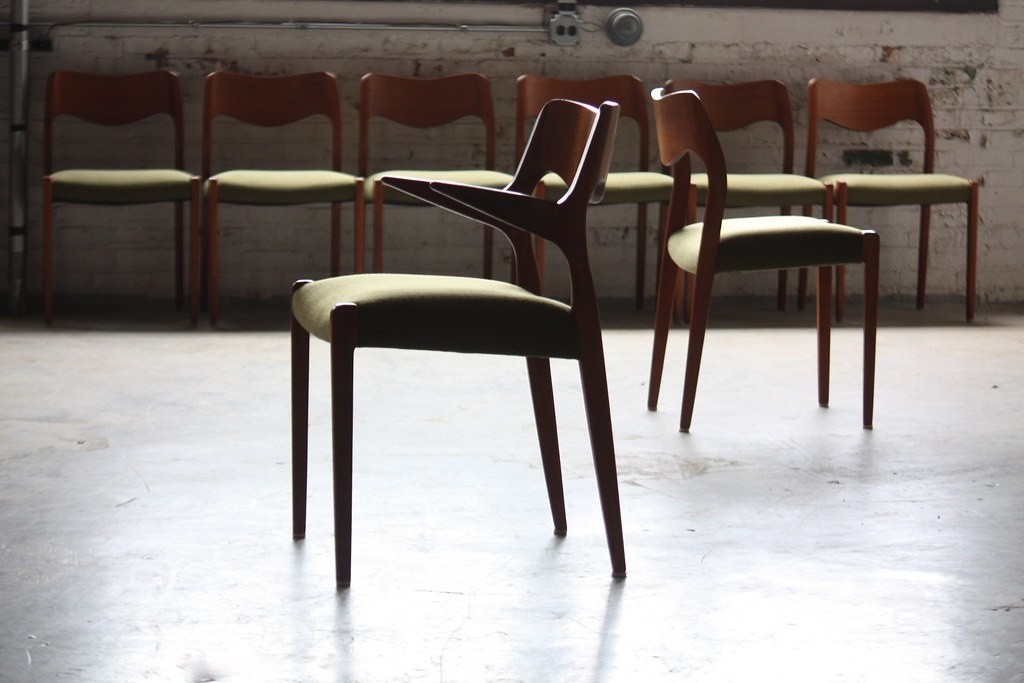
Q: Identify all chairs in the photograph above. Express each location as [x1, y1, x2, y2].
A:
[43, 66, 200, 326]
[648, 81, 880, 432]
[358, 70, 520, 289]
[289, 100, 628, 582]
[665, 78, 811, 325]
[515, 74, 682, 327]
[200, 69, 366, 326]
[808, 79, 981, 324]
